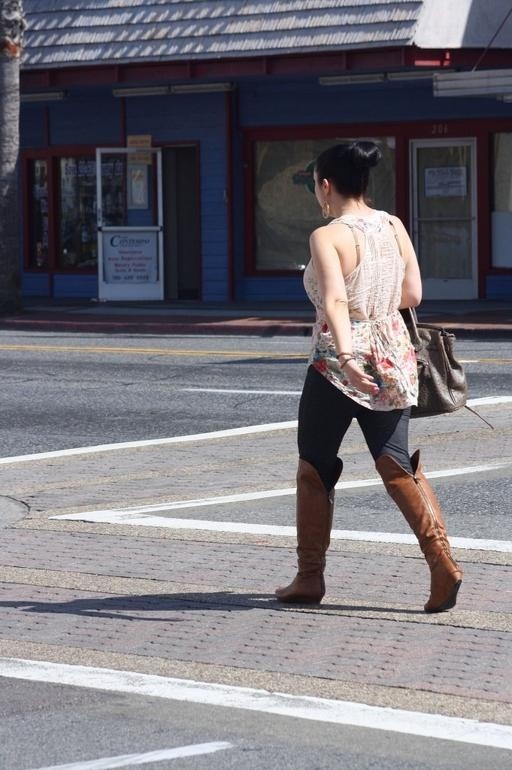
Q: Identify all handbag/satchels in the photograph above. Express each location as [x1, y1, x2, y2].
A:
[404, 307, 467, 419]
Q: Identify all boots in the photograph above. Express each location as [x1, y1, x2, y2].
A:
[372, 449, 463, 611]
[274, 458, 343, 601]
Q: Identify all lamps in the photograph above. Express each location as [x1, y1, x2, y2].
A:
[20, 90, 67, 102]
[319, 68, 457, 87]
[112, 81, 236, 97]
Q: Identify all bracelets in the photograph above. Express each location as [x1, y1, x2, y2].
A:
[340, 357, 357, 371]
[335, 352, 353, 361]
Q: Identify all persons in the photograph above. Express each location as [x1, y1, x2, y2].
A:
[267, 140, 465, 614]
